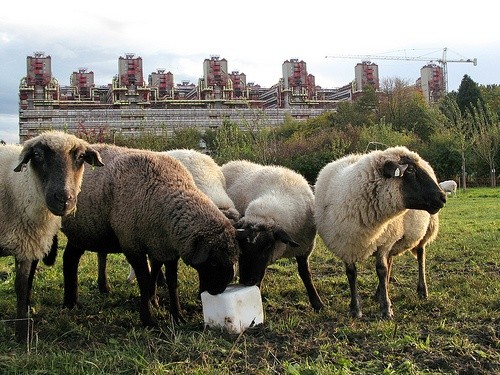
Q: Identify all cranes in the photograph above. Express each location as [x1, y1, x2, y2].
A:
[321, 47, 477, 98]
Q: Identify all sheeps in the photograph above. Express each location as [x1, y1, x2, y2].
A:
[0, 128, 107, 348]
[60, 140, 241, 335]
[439, 179, 458, 196]
[312, 143, 448, 321]
[158, 148, 241, 225]
[218, 158, 327, 316]
